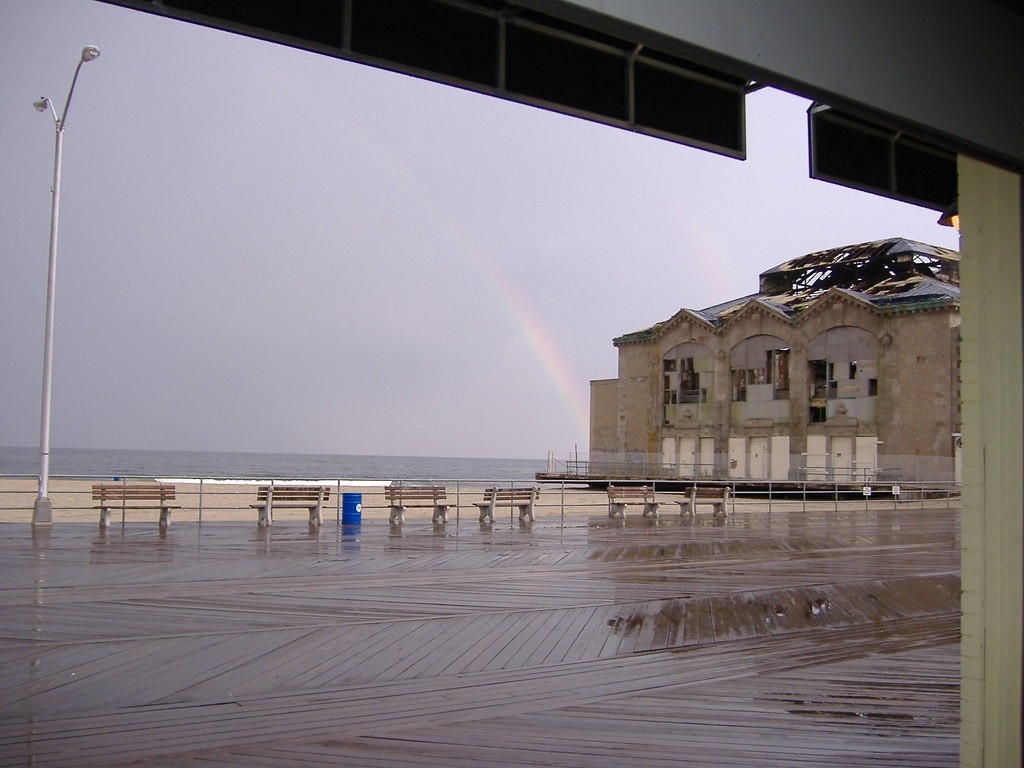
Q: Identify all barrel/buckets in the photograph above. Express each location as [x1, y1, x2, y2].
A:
[342, 493, 362, 525]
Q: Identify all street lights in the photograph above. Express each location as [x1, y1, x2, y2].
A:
[31, 43, 102, 531]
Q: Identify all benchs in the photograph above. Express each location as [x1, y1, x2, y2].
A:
[607, 485, 665, 518]
[673, 487, 731, 517]
[472, 487, 541, 522]
[248, 486, 330, 526]
[92, 485, 183, 527]
[385, 486, 456, 523]
